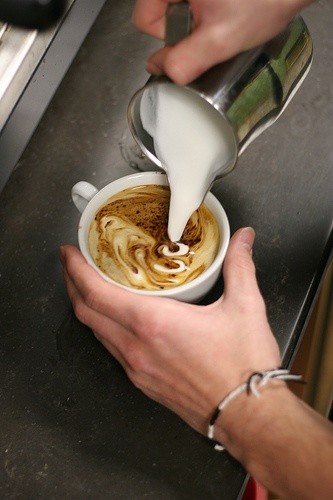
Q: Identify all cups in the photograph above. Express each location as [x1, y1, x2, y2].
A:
[126, 0, 313, 181]
[69, 171, 233, 303]
